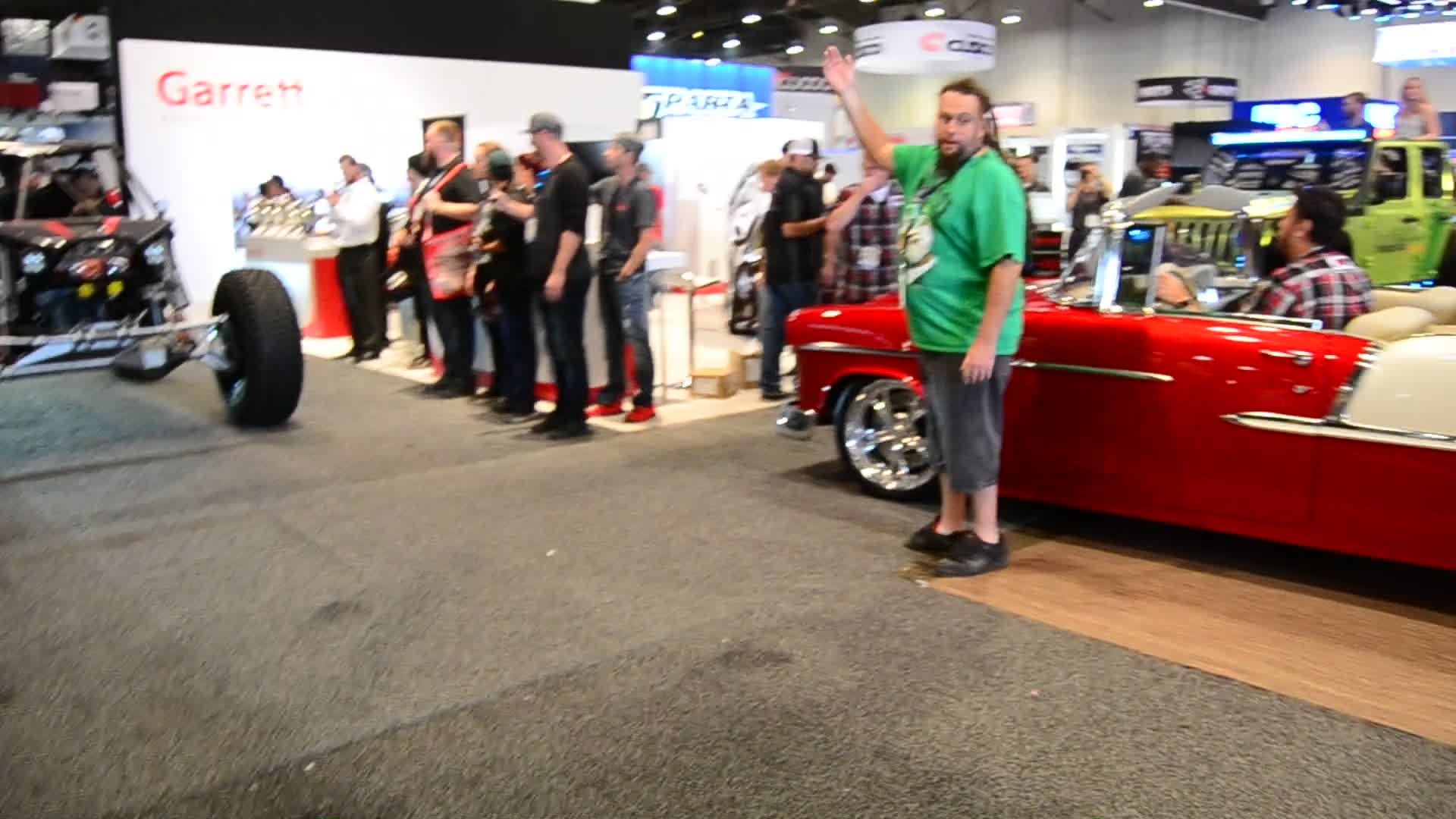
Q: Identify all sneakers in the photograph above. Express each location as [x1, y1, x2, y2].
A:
[623, 403, 658, 425]
[910, 515, 968, 556]
[585, 399, 624, 419]
[933, 530, 1010, 576]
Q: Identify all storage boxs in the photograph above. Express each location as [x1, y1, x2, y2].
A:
[689, 367, 736, 400]
[731, 348, 767, 389]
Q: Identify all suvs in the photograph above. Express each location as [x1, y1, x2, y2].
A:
[1115, 132, 1455, 288]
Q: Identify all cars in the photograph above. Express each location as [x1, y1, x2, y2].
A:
[0, 138, 303, 429]
[773, 214, 1456, 574]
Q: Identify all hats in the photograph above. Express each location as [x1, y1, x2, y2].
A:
[783, 131, 825, 161]
[522, 112, 560, 133]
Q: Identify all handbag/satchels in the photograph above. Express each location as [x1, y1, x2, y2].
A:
[424, 222, 482, 300]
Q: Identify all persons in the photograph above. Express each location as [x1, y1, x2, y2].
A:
[585, 130, 661, 425]
[1154, 184, 1373, 334]
[630, 161, 667, 250]
[1065, 166, 1113, 271]
[330, 154, 381, 364]
[816, 41, 1031, 582]
[1339, 91, 1376, 139]
[243, 173, 292, 231]
[419, 119, 480, 403]
[1117, 152, 1159, 197]
[1392, 76, 1438, 141]
[516, 111, 595, 441]
[709, 126, 1057, 405]
[389, 147, 436, 372]
[513, 148, 545, 201]
[356, 162, 390, 350]
[461, 137, 538, 415]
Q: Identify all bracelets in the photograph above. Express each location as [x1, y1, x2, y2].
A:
[1171, 296, 1199, 310]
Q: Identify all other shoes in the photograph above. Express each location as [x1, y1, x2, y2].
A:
[405, 353, 431, 368]
[530, 416, 595, 443]
[473, 391, 536, 414]
[347, 345, 379, 361]
[424, 379, 475, 399]
[762, 386, 793, 401]
